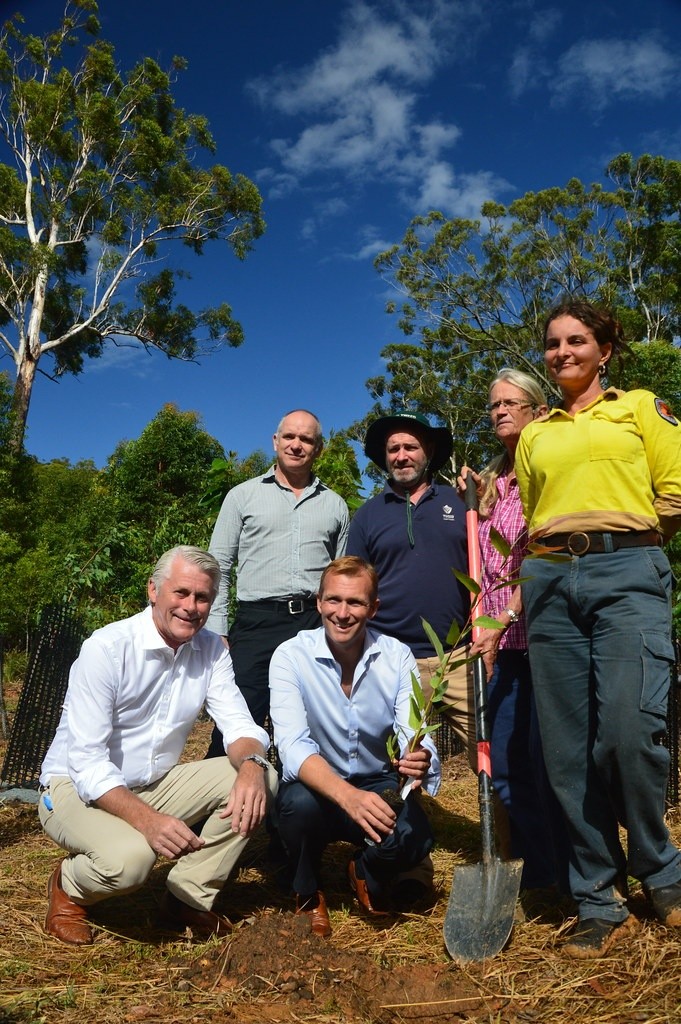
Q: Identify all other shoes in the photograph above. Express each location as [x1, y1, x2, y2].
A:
[480, 820, 509, 843]
[646, 878, 681, 927]
[559, 917, 615, 957]
[257, 817, 285, 860]
[520, 883, 561, 919]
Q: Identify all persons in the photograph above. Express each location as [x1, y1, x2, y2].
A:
[516, 302, 681, 958]
[40, 545, 279, 941]
[202, 410, 351, 755]
[457, 369, 571, 915]
[345, 412, 479, 778]
[269, 557, 441, 935]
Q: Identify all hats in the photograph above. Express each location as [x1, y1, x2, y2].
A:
[366, 411, 452, 474]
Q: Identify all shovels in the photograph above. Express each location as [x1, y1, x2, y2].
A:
[442, 469, 525, 963]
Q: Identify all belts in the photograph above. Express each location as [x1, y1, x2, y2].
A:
[39, 785, 49, 791]
[532, 530, 660, 555]
[238, 599, 317, 615]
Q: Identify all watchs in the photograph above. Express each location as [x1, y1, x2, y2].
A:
[504, 608, 519, 622]
[243, 753, 268, 772]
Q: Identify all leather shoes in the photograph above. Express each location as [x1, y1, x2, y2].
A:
[179, 901, 239, 940]
[345, 858, 392, 922]
[45, 856, 94, 948]
[296, 887, 330, 937]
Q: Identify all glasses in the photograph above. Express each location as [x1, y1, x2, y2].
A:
[485, 400, 535, 414]
